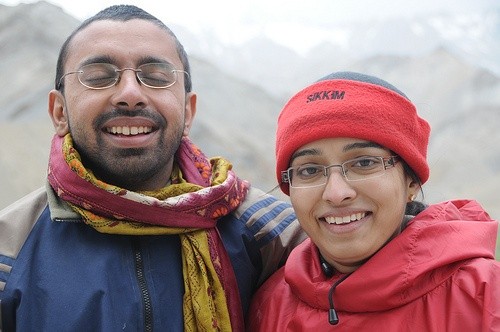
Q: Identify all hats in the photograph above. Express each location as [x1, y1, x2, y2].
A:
[275, 70, 430, 197]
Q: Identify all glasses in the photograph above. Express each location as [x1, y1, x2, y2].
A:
[280, 155, 400, 189]
[57, 62, 189, 94]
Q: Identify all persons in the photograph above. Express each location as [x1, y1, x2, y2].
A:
[0, 5, 311, 332]
[245, 72, 500, 332]
